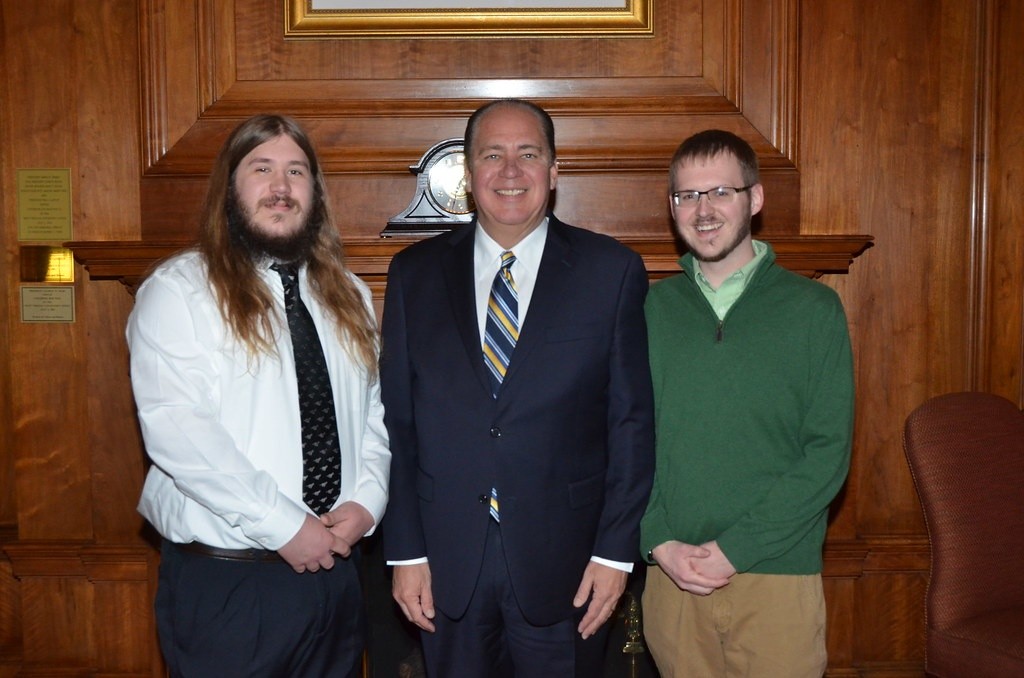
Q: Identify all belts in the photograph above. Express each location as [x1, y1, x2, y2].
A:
[154, 535, 364, 563]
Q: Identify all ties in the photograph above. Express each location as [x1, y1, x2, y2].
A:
[482, 251, 518, 522]
[272, 256, 341, 518]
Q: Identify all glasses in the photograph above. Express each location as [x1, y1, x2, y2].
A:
[670, 183, 753, 207]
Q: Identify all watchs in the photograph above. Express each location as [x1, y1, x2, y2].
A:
[648, 550, 652, 560]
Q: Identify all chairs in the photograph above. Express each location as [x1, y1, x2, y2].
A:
[900, 390, 1024, 678]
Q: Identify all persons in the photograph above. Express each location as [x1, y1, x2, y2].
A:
[381, 107, 656, 678]
[125, 113, 391, 678]
[641, 128, 855, 678]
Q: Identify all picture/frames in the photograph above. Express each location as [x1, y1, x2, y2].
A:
[283, 0, 655, 40]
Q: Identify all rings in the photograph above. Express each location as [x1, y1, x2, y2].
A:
[611, 608, 616, 612]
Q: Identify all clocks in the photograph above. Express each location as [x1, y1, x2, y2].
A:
[380, 137, 477, 238]
